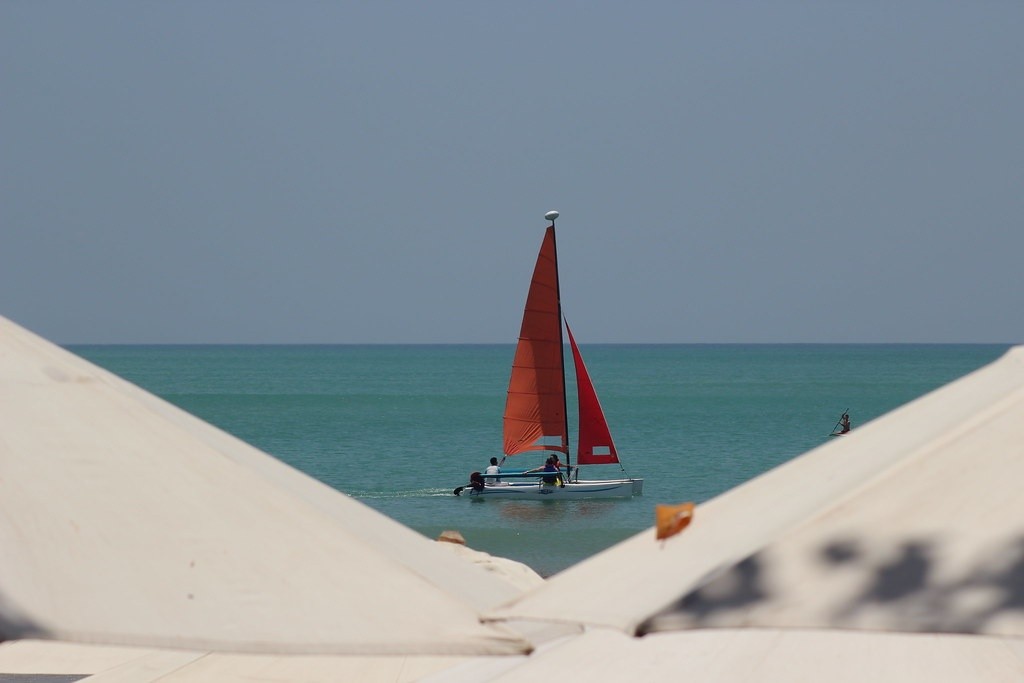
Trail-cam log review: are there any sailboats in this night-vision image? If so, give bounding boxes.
[453,211,645,498]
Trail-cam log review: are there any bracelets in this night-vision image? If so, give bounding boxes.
[527,471,528,473]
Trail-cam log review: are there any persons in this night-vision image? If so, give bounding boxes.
[839,415,850,433]
[523,454,576,486]
[484,457,501,484]
